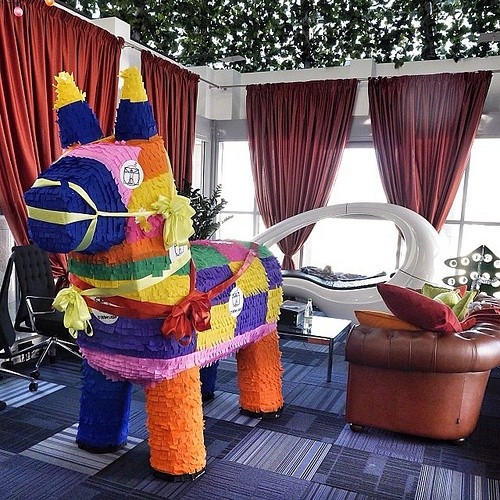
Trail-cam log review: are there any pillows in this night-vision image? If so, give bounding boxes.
[433,290,462,308]
[460,316,477,330]
[421,282,449,298]
[452,289,480,321]
[377,282,464,333]
[455,284,468,295]
[353,309,422,330]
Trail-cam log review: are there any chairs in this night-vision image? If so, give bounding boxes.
[0,243,84,392]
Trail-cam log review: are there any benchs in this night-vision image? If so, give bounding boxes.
[250,201,441,325]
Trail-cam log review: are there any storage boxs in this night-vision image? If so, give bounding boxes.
[278,298,307,326]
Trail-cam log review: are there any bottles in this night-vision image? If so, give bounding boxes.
[303,298,312,329]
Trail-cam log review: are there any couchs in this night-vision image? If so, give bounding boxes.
[344,289,500,446]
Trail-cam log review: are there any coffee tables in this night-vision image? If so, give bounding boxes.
[276,315,352,383]
